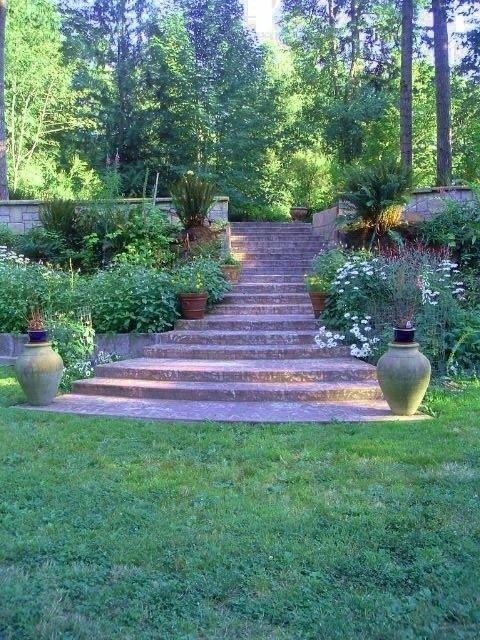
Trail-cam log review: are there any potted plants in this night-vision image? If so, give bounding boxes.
[26,306,48,343]
[177,266,207,321]
[388,301,418,342]
[220,256,241,285]
[309,248,347,319]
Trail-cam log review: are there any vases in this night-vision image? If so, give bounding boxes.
[289,206,309,222]
